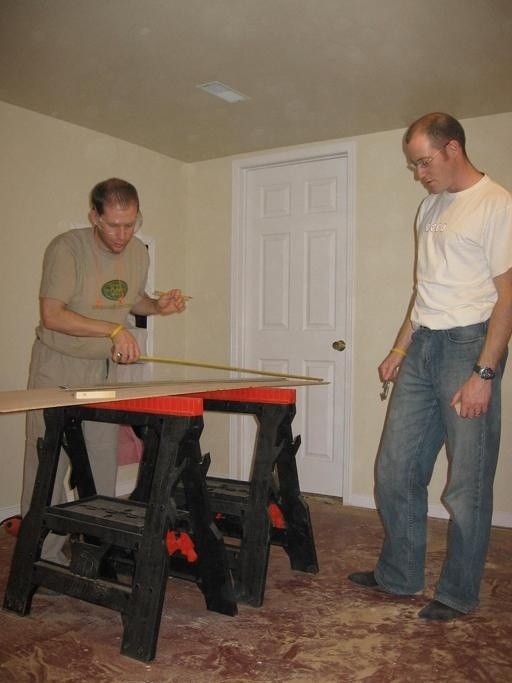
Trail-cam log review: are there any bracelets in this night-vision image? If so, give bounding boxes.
[391,346,406,358]
[110,324,126,340]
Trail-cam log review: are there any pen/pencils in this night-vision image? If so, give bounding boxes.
[153,290,193,302]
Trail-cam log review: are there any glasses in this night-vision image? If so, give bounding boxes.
[93,206,144,236]
[406,141,448,171]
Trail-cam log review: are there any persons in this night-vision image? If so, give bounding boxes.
[22,178,187,596]
[348,111,512,621]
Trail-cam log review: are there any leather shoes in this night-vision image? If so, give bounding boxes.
[347,569,378,587]
[416,600,466,620]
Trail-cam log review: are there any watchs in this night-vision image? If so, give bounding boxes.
[473,363,496,380]
